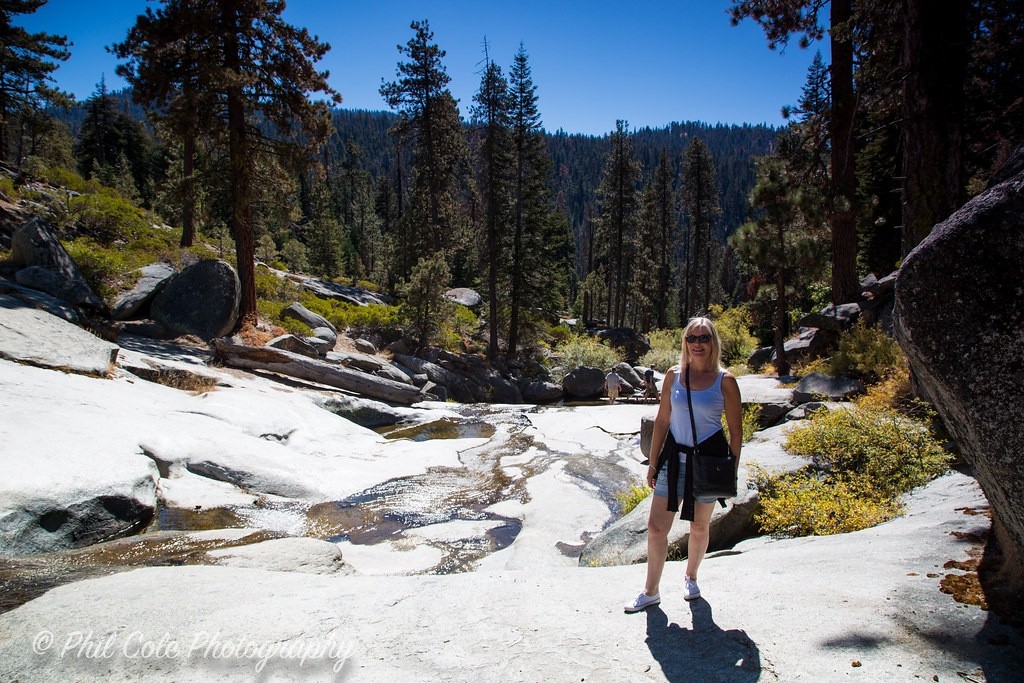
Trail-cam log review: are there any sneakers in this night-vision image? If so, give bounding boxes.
[683,576,701,600]
[623,587,662,612]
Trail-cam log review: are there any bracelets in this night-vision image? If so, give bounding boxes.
[649,465,655,469]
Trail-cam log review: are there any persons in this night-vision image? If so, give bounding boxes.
[605,368,622,404]
[644,364,660,401]
[623,317,744,612]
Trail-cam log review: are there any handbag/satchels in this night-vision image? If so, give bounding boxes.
[691,454,738,498]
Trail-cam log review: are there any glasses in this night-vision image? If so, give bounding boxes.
[685,335,713,343]
